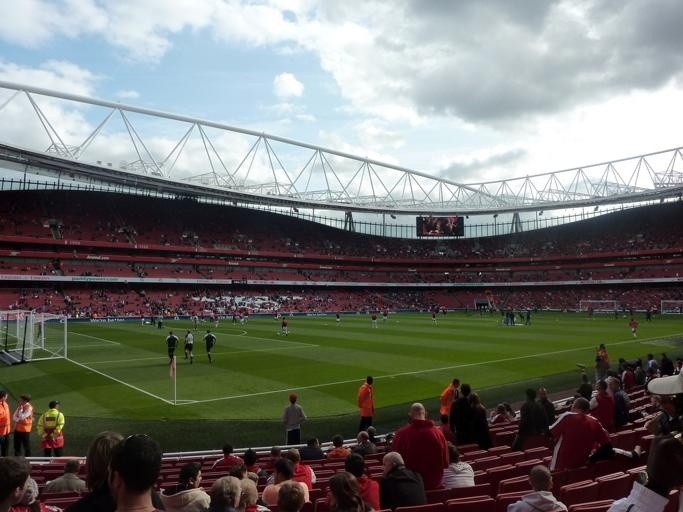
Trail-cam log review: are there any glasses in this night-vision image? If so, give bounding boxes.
[574,394,581,399]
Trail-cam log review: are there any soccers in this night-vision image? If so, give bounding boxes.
[276,332,280,335]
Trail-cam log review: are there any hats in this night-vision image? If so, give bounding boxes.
[576,384,593,401]
[647,365,683,395]
[48,401,59,409]
[289,393,297,401]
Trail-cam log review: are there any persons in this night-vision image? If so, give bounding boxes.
[164,330,178,363]
[594,344,610,378]
[5,217,683,328]
[1,350,682,512]
[281,316,287,336]
[627,317,639,338]
[201,329,216,363]
[182,328,194,364]
